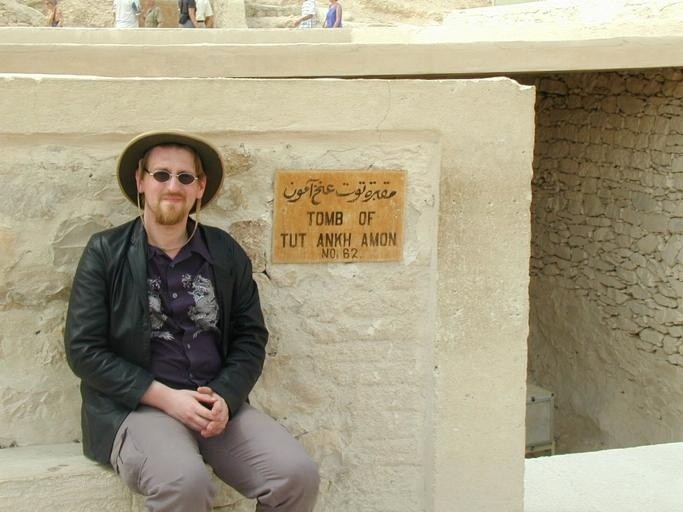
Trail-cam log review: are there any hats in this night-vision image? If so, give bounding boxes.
[117,129,223,212]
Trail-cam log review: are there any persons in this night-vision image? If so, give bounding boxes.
[177,0,200,27]
[112,0,142,28]
[292,0,319,28]
[323,0,342,28]
[143,0,166,27]
[195,0,214,28]
[61,131,320,512]
[45,0,64,28]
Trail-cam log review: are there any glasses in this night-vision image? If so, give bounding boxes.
[144,167,201,185]
